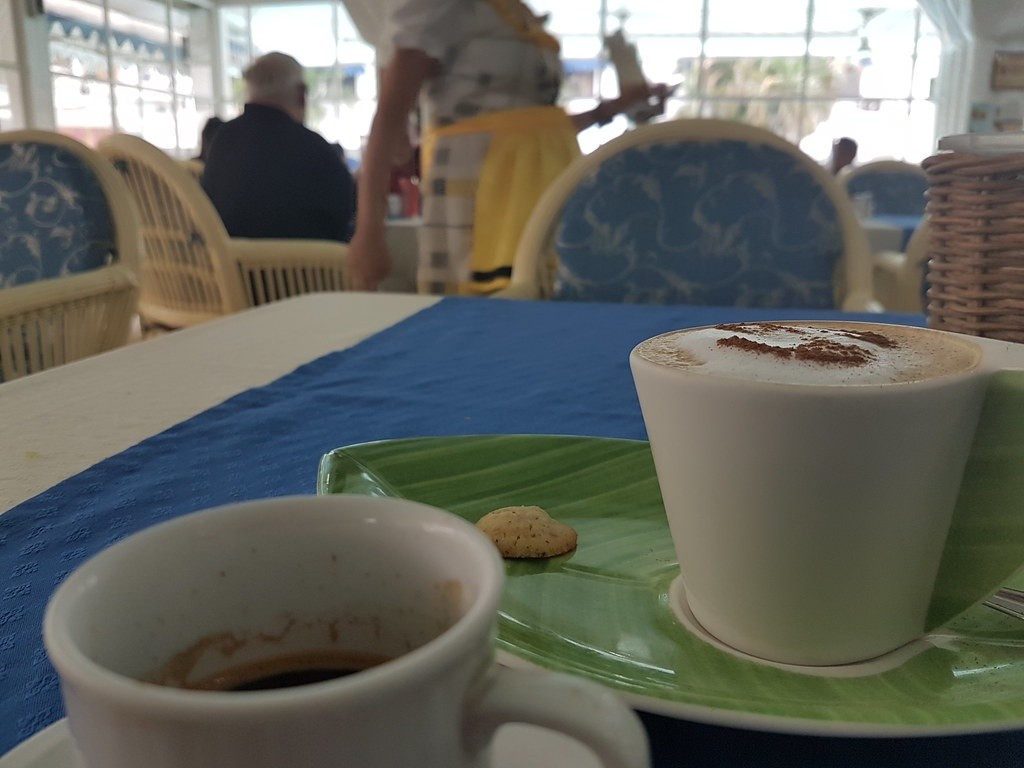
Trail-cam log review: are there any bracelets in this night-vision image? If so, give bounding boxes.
[593,103,613,127]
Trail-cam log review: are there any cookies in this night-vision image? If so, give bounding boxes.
[473,506,578,558]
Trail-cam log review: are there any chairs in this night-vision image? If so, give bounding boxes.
[0,118,938,383]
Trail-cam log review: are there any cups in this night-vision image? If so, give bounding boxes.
[42,493,654,767]
[628,319,985,666]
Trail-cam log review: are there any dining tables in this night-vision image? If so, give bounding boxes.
[0,293,1023,768]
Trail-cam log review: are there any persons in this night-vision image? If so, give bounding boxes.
[191,53,420,305]
[344,0,681,299]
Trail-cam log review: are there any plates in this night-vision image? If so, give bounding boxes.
[311,428,1022,739]
[0,644,605,765]
